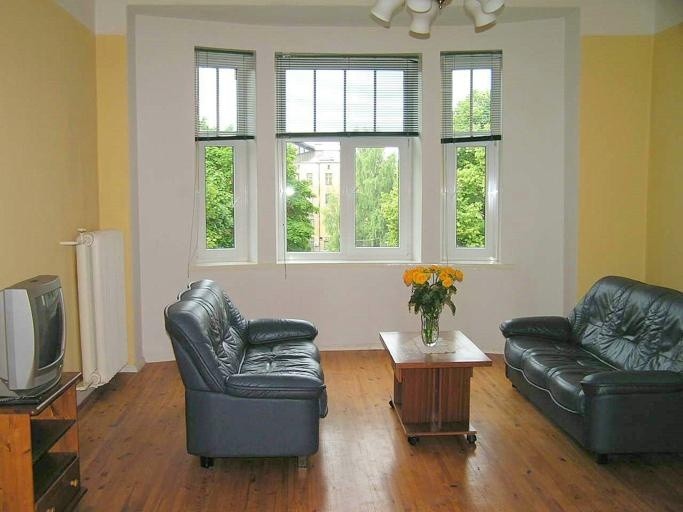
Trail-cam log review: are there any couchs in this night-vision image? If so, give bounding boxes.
[497,272,682,466]
[164,275,331,471]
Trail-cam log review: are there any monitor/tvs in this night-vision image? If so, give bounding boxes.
[0,274,68,399]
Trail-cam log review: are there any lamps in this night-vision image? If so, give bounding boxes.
[366,0,506,41]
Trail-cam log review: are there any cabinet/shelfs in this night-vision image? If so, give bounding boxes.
[0,369,89,512]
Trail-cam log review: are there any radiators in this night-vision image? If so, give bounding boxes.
[55,226,128,394]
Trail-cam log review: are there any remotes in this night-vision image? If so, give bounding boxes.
[0,396,42,405]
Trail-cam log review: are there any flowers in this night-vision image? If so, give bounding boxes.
[399,263,463,344]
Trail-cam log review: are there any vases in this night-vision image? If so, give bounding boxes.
[416,307,442,348]
